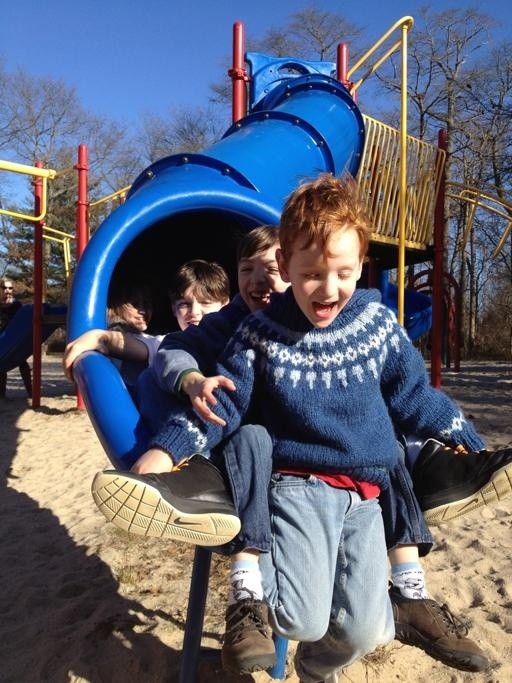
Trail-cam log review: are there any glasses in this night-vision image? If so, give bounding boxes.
[0,286,13,289]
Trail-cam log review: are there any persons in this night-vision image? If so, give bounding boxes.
[62,170,512,683]
[1,275,35,404]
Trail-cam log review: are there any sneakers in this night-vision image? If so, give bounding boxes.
[411,438,512,527]
[389,581,488,672]
[222,592,277,676]
[91,453,241,547]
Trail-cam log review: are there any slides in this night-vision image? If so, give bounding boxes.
[67,51,440,473]
